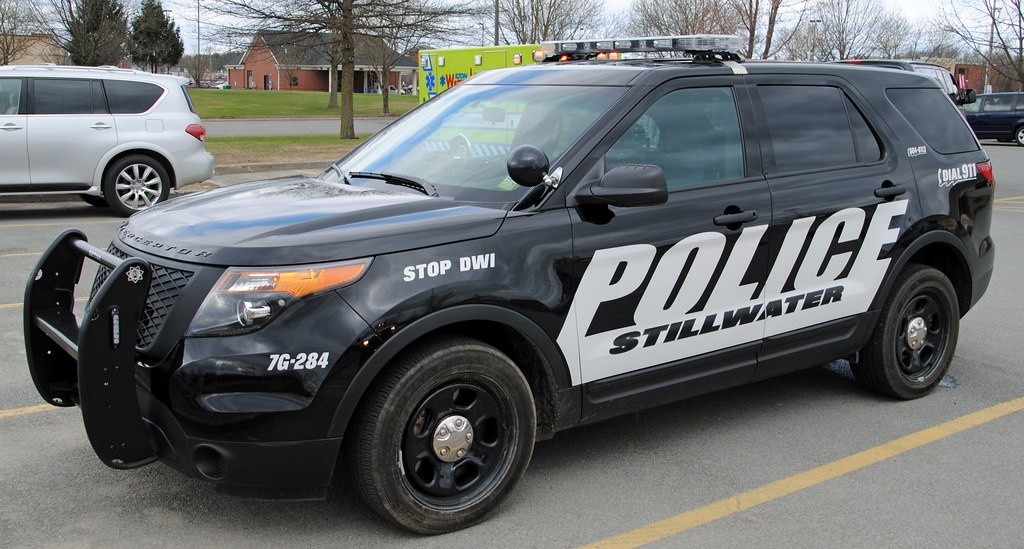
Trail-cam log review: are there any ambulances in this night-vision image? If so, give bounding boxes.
[417,45,658,192]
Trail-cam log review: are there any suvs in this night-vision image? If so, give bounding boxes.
[0,63,214,217]
[23,35,996,536]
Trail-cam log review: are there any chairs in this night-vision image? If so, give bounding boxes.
[515,108,563,159]
[660,105,718,183]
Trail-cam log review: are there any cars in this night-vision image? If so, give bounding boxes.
[963,92,1024,146]
